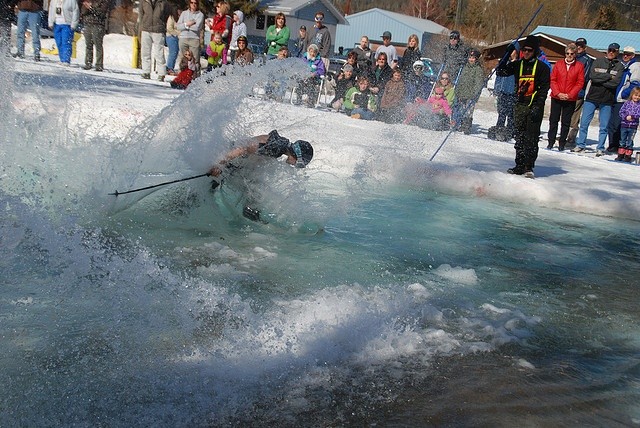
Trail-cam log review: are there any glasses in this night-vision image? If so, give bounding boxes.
[291,143,306,168]
[565,53,574,55]
[450,35,458,39]
[190,2,197,4]
[523,48,534,52]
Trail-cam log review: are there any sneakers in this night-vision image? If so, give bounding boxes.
[571,146,584,152]
[507,164,525,175]
[596,150,606,157]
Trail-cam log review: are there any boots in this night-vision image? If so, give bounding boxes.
[624,149,633,161]
[615,148,626,161]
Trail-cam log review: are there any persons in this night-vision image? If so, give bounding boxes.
[367,51,393,106]
[81,0,108,71]
[455,47,483,134]
[227,10,247,50]
[165,4,181,75]
[303,11,331,57]
[206,31,227,71]
[487,49,517,142]
[170,49,196,89]
[547,42,585,150]
[431,71,455,115]
[344,78,379,119]
[331,63,356,111]
[406,59,431,125]
[379,68,406,123]
[496,34,550,177]
[205,1,233,49]
[399,33,422,78]
[176,0,204,76]
[374,30,399,68]
[156,129,314,225]
[12,0,43,61]
[302,43,326,84]
[47,0,80,68]
[414,87,451,129]
[290,24,306,57]
[564,37,591,146]
[139,0,167,81]
[441,29,469,86]
[333,51,367,83]
[571,42,625,156]
[269,46,289,59]
[234,35,254,66]
[606,45,640,151]
[614,87,640,162]
[351,35,374,66]
[266,12,290,60]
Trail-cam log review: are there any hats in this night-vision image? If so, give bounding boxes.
[608,43,620,51]
[623,46,635,54]
[576,38,586,45]
[413,60,424,71]
[300,26,306,31]
[523,35,539,49]
[380,31,391,37]
[237,36,247,41]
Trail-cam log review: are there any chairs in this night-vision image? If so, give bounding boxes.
[423,64,462,129]
[290,56,330,109]
